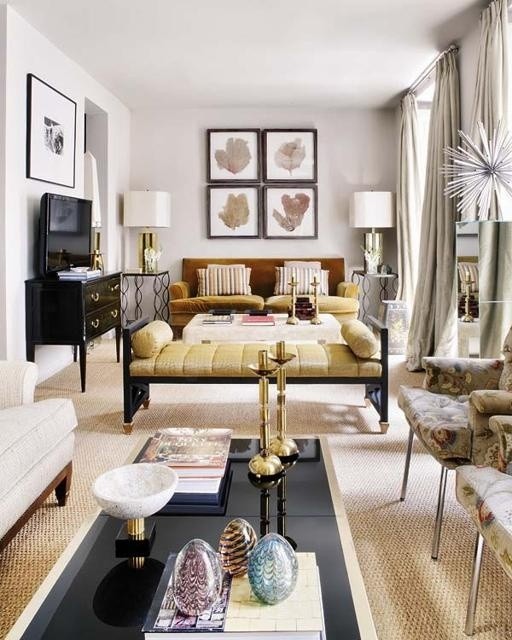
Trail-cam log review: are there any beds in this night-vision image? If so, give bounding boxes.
[122,315,390,435]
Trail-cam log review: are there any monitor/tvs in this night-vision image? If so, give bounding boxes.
[38,192,93,277]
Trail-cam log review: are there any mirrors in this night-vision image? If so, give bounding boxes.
[455,221,479,324]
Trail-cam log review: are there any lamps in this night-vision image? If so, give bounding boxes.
[349,187,396,275]
[122,187,170,273]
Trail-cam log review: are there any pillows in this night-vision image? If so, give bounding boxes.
[196,268,253,296]
[206,264,245,269]
[457,263,479,293]
[273,267,329,296]
[283,260,322,269]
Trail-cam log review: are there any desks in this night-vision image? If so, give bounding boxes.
[120,266,170,336]
[351,269,398,327]
[23,271,122,393]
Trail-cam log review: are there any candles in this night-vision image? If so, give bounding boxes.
[465,270,470,281]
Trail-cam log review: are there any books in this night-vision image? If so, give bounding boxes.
[150,458,233,517]
[139,427,233,493]
[202,315,232,324]
[242,315,275,326]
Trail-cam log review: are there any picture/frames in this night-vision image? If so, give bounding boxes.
[263,184,319,240]
[262,128,318,184]
[25,72,77,190]
[208,127,263,184]
[206,184,263,239]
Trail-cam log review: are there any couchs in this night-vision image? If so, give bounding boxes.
[0,360,79,552]
[166,257,361,342]
[457,255,478,306]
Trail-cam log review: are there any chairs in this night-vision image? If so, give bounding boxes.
[397,327,512,560]
[454,415,512,635]
